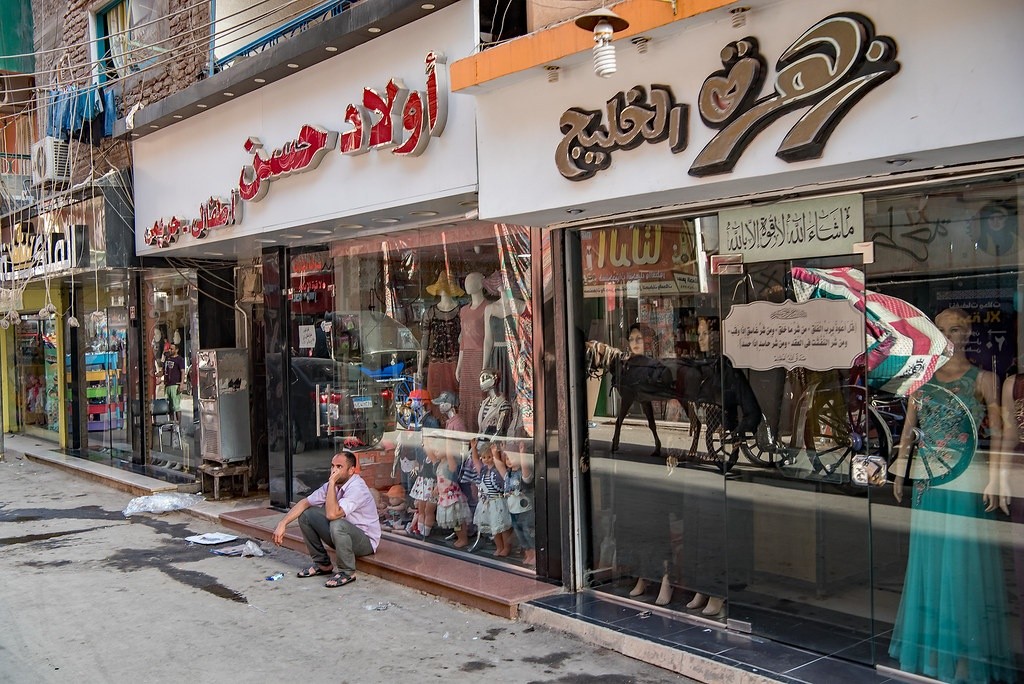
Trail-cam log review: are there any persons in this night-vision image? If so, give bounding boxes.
[26,374,47,427]
[155,344,185,431]
[272,451,381,588]
[612,307,1024,684]
[977,206,1024,257]
[369,271,536,565]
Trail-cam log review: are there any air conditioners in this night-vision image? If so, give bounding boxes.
[29,136,72,190]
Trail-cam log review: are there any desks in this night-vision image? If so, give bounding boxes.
[747,470,902,599]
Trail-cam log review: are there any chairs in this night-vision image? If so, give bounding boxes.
[151,398,182,452]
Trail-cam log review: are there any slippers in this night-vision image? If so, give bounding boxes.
[324,572,356,587]
[297,565,332,578]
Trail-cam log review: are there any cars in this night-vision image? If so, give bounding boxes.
[290,356,398,454]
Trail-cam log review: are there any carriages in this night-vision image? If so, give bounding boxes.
[584,337,978,497]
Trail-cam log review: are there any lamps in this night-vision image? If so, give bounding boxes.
[547,67,558,82]
[730,8,747,28]
[636,39,648,54]
[574,0,629,79]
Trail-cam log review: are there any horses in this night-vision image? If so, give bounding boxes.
[585,341,713,457]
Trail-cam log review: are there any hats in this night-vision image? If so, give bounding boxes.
[382,485,406,501]
[426,270,465,297]
[431,391,460,407]
[409,389,430,404]
[481,269,530,299]
